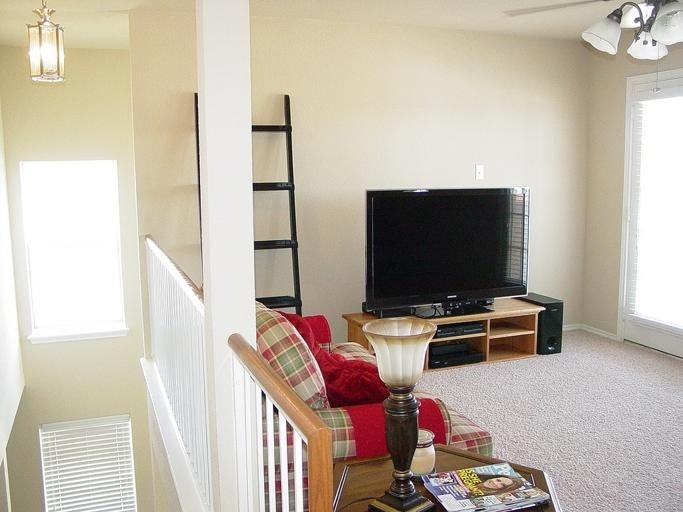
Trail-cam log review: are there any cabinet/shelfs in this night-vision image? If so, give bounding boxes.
[341,297,545,372]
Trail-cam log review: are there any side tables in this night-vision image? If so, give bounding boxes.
[331,443,561,512]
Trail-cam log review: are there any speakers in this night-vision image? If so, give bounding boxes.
[514,292,564,355]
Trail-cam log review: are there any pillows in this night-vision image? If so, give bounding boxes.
[255,299,387,409]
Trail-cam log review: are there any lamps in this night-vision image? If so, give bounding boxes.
[360,317,438,512]
[580,0,682,61]
[24,1,65,83]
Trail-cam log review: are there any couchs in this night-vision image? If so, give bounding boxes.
[195,285,492,512]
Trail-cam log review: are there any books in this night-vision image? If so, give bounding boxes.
[421,461,550,512]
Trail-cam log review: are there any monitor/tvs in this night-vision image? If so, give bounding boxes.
[364,187,530,318]
